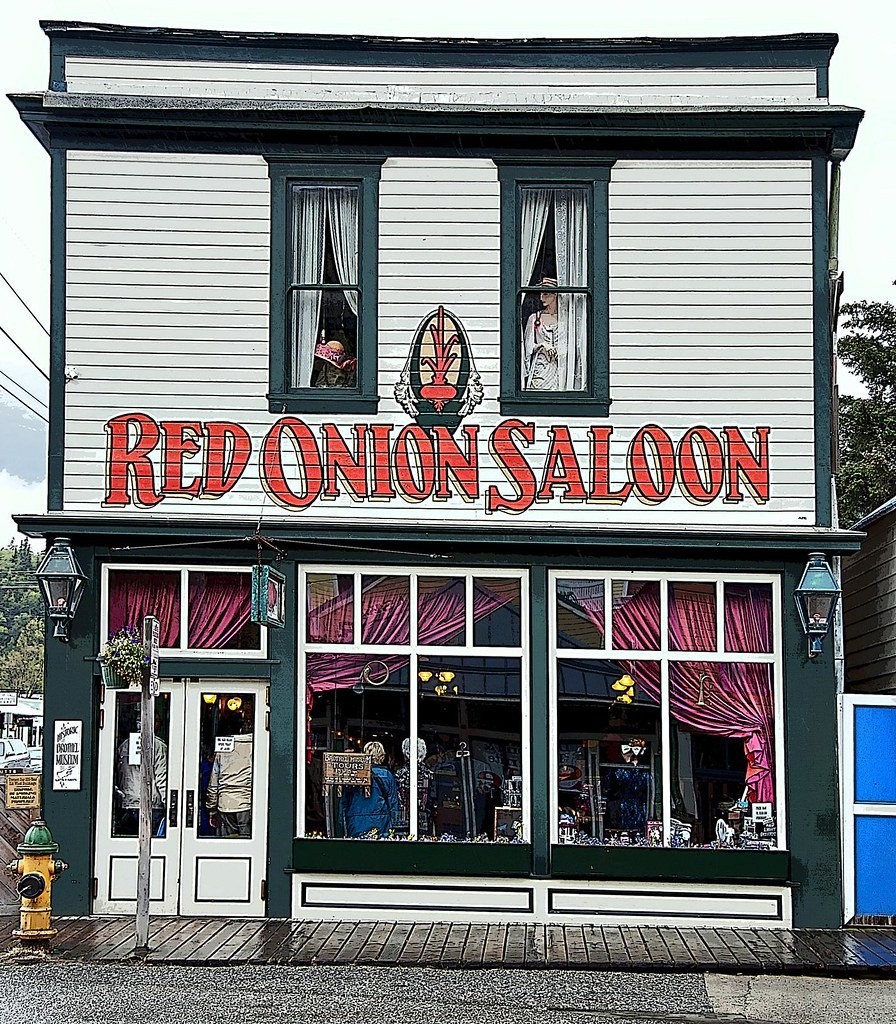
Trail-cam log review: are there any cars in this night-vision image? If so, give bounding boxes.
[27,747,43,774]
[0,739,31,768]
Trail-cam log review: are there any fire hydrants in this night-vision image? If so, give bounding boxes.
[6,818,68,945]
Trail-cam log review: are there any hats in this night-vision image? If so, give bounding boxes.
[314,341,357,371]
[537,278,557,288]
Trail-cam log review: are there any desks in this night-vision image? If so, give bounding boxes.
[493,806,521,841]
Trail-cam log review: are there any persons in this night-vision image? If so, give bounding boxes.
[524,276,561,390]
[601,738,652,839]
[114,708,168,835]
[313,339,357,387]
[337,741,399,839]
[394,736,436,836]
[205,714,254,835]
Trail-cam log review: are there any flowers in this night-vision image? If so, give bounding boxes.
[97,624,150,687]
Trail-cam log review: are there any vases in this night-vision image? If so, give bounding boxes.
[99,662,130,689]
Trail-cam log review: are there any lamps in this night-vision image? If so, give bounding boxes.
[418,671,457,696]
[611,675,635,704]
[792,553,843,659]
[33,537,92,644]
